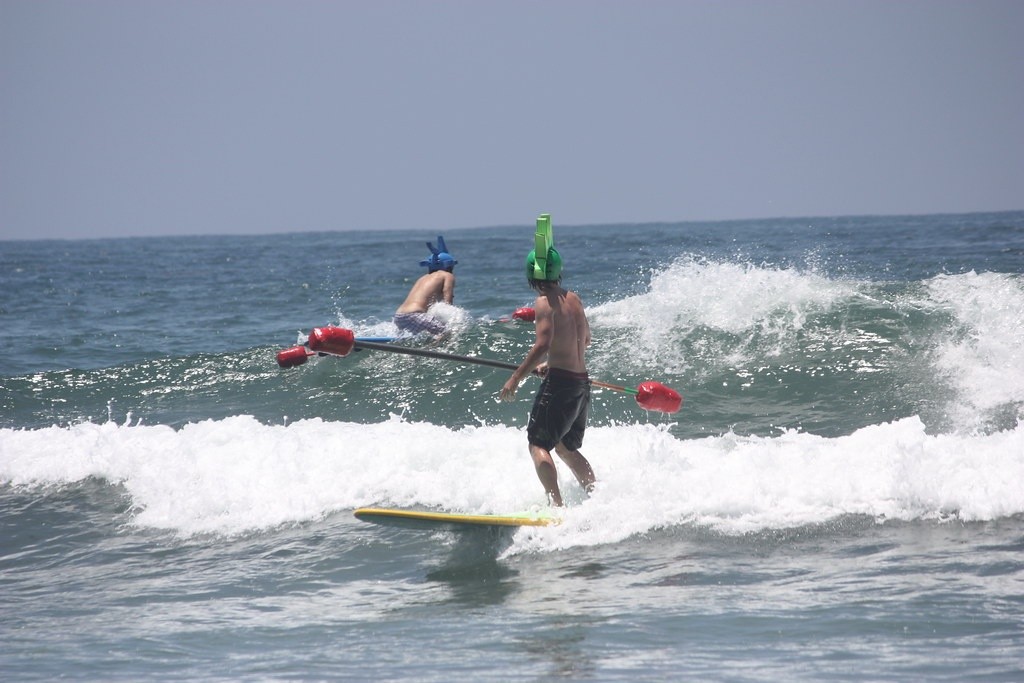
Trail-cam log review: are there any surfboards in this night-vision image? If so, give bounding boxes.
[301,335,399,347]
[352,506,549,532]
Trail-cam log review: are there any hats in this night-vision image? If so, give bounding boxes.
[419,235,458,270]
[526,214,561,281]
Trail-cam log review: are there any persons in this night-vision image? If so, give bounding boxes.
[499,214,599,509]
[394,236,457,339]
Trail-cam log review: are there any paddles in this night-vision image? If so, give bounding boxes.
[276,306,537,369]
[309,325,682,413]
